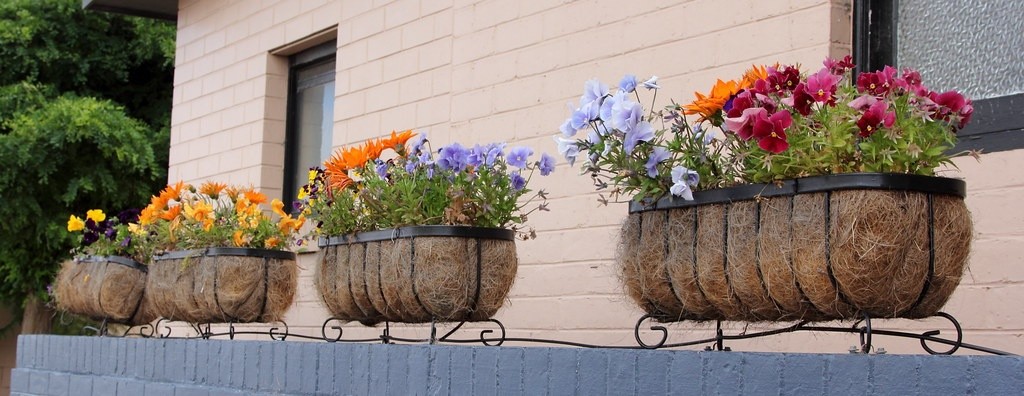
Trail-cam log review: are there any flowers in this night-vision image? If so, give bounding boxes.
[297,131,558,246]
[136,179,299,271]
[67,209,149,266]
[552,55,986,206]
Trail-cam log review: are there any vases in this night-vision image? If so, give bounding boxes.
[146,248,304,340]
[48,254,155,338]
[617,171,974,355]
[314,224,519,347]
